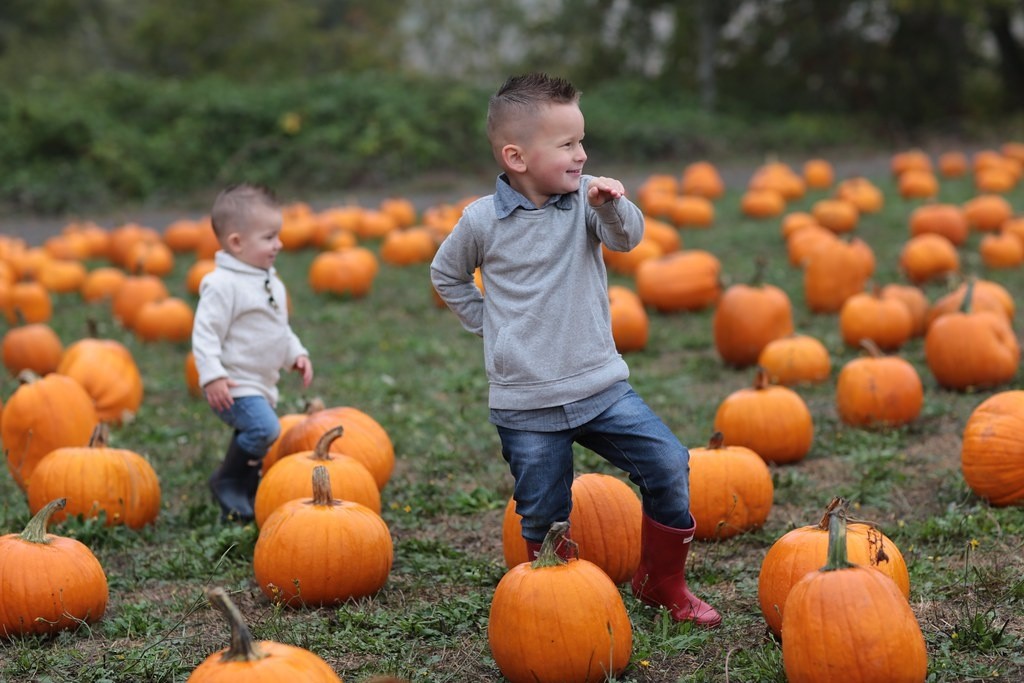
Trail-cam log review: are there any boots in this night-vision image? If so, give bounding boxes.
[631,511,722,628]
[210,429,257,518]
[527,530,569,562]
[220,459,262,523]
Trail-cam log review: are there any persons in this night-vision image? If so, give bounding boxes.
[431,74,722,631]
[192,181,313,521]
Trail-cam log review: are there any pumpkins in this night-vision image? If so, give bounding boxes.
[0,146,1024,683]
